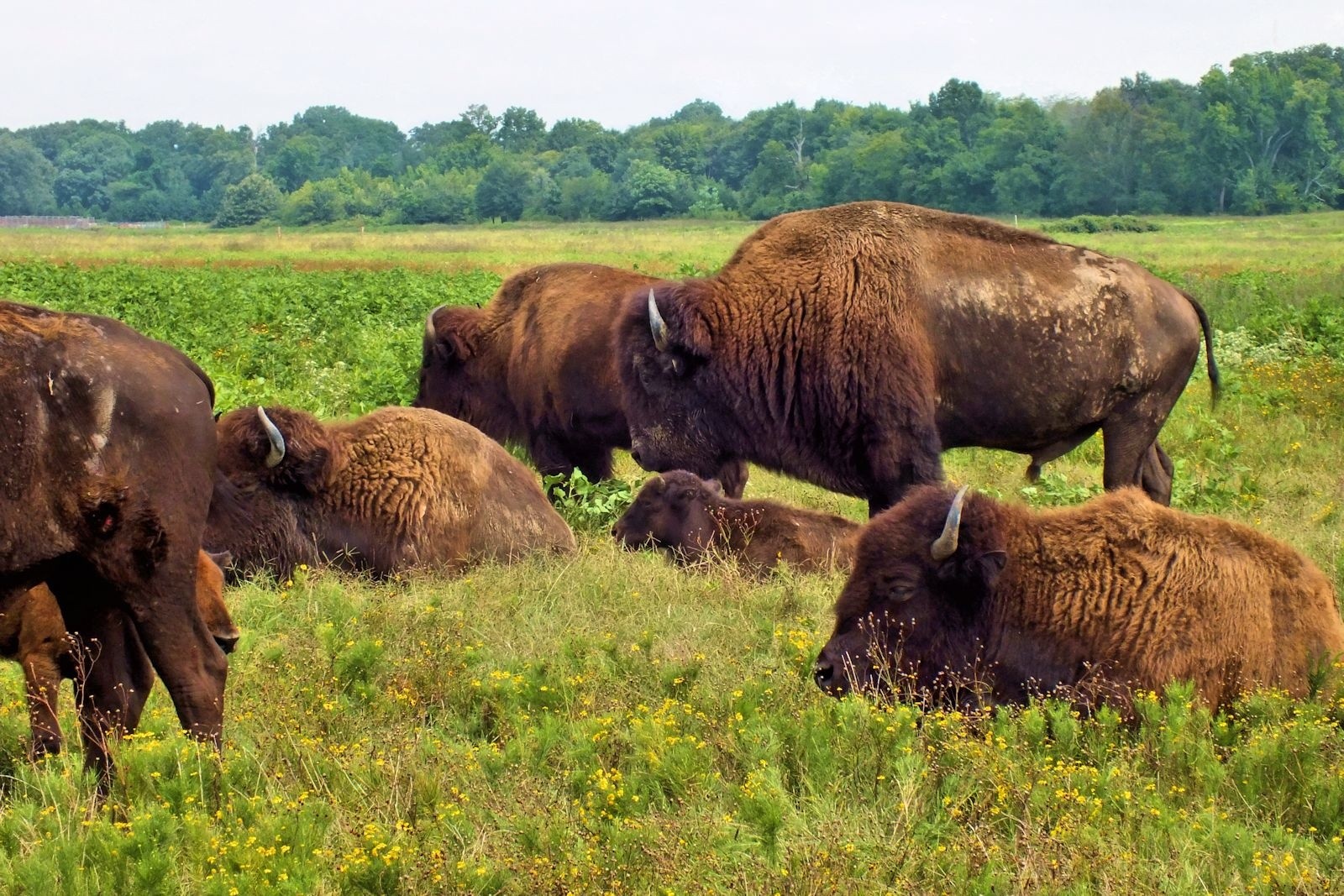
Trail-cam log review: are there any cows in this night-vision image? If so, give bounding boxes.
[611,470,866,576]
[0,302,578,825]
[414,261,673,520]
[611,202,1226,504]
[813,485,1344,721]
